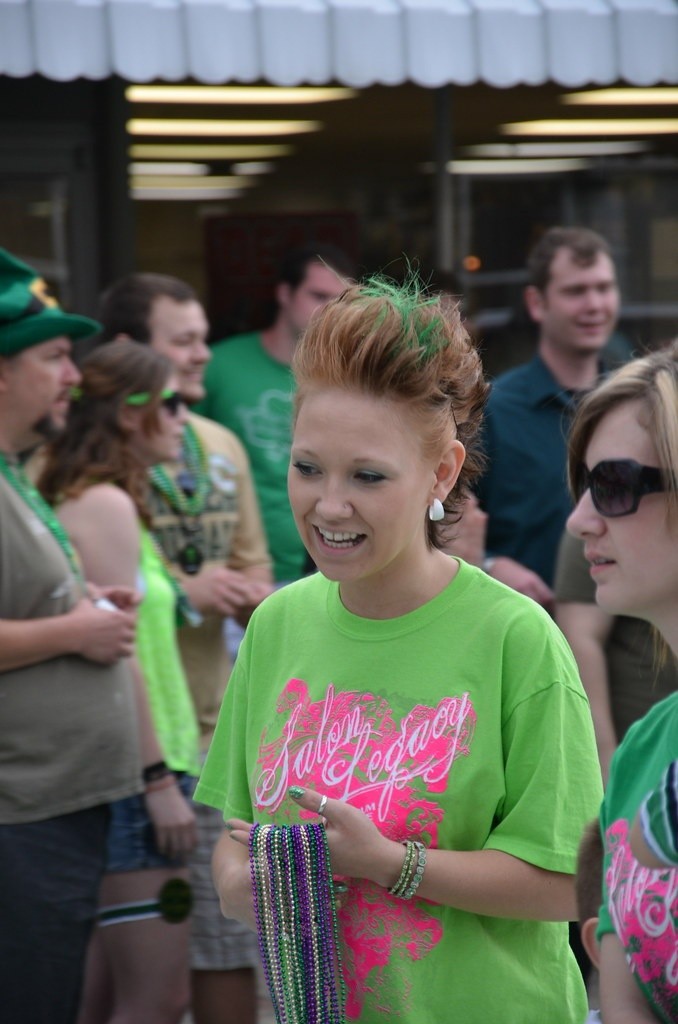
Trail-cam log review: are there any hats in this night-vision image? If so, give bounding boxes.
[0,249,104,356]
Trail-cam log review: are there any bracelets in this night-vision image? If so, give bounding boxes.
[388,841,427,900]
[482,556,496,572]
[143,761,175,793]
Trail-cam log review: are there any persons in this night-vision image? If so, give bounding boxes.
[27,347,207,1024]
[551,506,678,789]
[191,285,603,1024]
[563,342,678,1024]
[105,273,274,1024]
[575,817,603,1024]
[0,250,148,1024]
[193,238,365,589]
[436,226,631,620]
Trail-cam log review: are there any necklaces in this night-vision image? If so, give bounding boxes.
[249,822,346,1023]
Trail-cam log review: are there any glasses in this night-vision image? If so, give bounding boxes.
[575,460,677,517]
[124,389,179,416]
[140,822,194,924]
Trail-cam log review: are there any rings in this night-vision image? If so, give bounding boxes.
[318,795,327,814]
